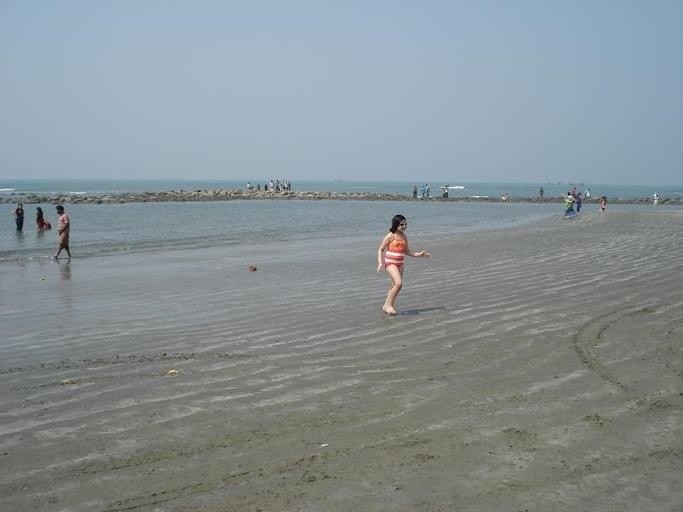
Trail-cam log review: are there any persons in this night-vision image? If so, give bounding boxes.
[53,205,71,259]
[35,207,51,231]
[376,214,430,315]
[536,186,607,219]
[412,184,449,199]
[247,179,291,192]
[12,203,24,232]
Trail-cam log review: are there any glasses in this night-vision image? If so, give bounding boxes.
[400,223,407,226]
